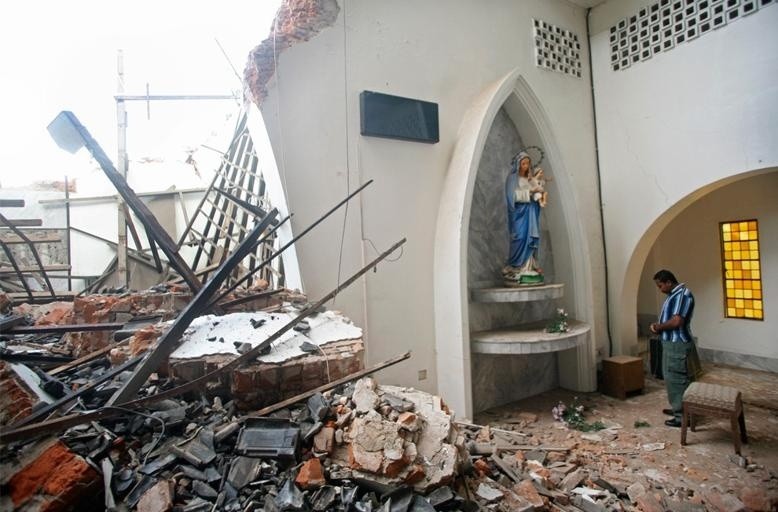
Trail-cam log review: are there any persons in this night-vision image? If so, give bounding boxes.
[504,151,542,274]
[527,166,554,208]
[647,269,695,427]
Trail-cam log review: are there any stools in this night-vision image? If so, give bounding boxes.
[680,381,749,455]
[601,354,646,401]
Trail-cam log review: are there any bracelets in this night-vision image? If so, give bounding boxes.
[650,322,657,333]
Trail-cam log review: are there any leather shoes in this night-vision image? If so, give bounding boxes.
[662,408,674,416]
[664,418,691,427]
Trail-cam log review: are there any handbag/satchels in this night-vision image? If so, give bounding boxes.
[649,338,664,380]
